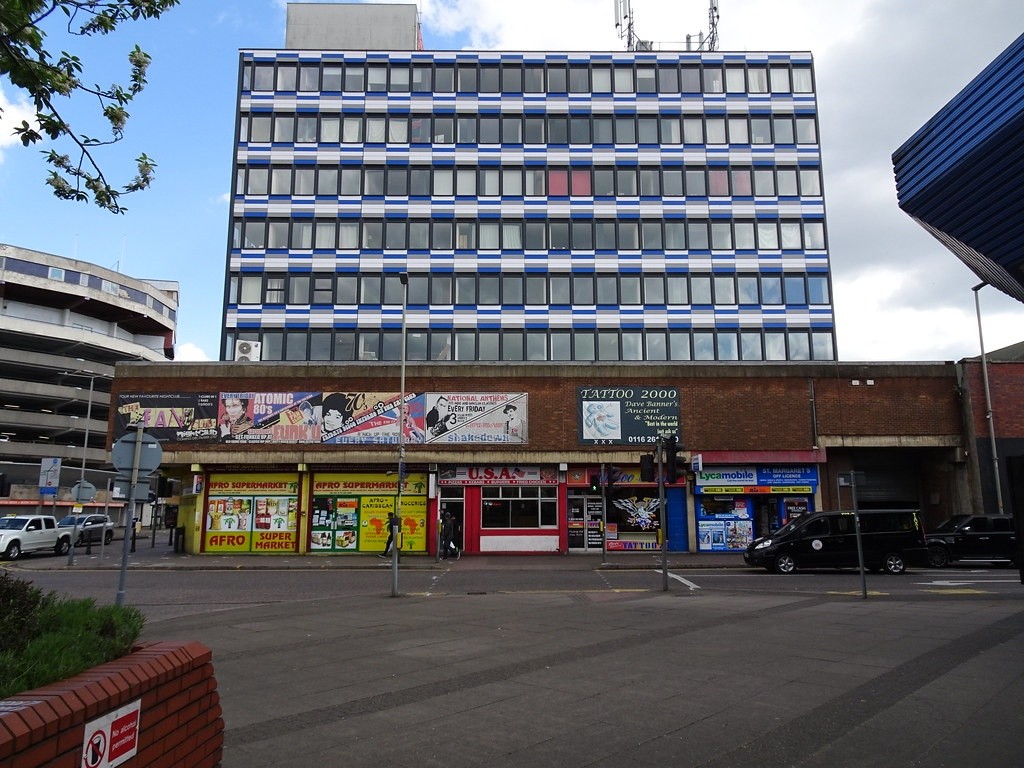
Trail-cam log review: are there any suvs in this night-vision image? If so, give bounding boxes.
[55,514,115,548]
[915,512,1017,570]
[743,507,930,576]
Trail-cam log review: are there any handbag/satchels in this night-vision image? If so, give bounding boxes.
[443,541,455,552]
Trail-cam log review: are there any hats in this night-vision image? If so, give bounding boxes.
[445,512,451,517]
[503,405,517,414]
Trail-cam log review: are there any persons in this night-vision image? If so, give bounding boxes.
[219,399,253,443]
[427,397,451,436]
[440,512,460,560]
[299,401,322,425]
[377,512,398,558]
[503,404,526,443]
[396,403,417,440]
[322,393,354,432]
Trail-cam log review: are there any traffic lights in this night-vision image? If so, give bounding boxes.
[664,434,689,484]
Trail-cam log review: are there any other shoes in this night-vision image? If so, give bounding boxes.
[455,551,460,560]
[377,554,386,559]
[439,556,447,561]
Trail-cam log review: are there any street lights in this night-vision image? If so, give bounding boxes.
[392,270,410,598]
[973,281,1004,516]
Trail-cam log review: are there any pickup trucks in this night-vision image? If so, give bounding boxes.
[0,514,82,561]
